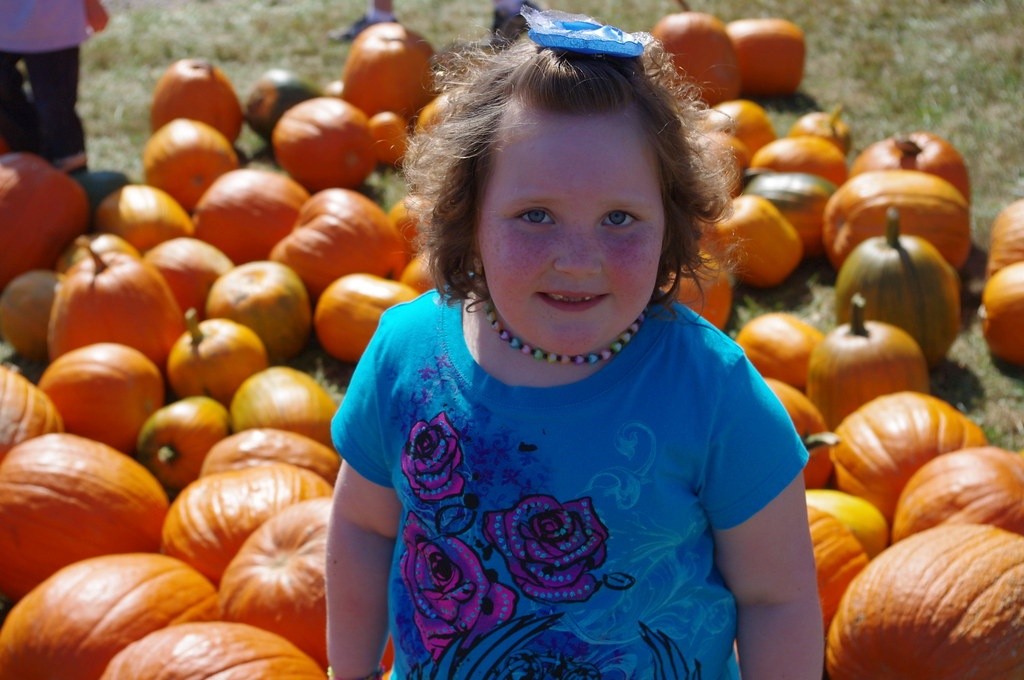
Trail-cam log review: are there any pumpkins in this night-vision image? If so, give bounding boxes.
[0,0,1024,680]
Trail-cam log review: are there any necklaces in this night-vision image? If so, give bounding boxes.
[484,300,648,364]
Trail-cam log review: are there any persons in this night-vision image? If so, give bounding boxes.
[0,0,110,173]
[325,4,828,680]
